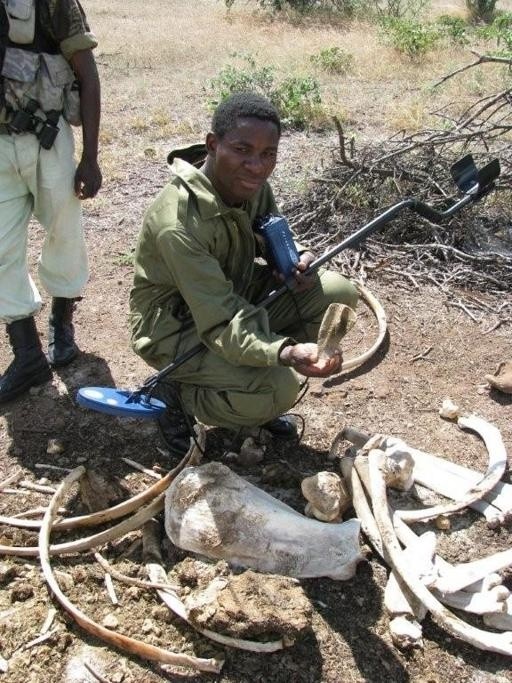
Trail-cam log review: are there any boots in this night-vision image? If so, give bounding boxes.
[144,376,197,455]
[47,297,78,367]
[258,416,299,439]
[0,315,53,403]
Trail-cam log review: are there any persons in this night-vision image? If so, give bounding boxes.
[130,94,358,456]
[0,0,102,400]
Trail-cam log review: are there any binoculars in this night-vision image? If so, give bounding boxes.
[9,100,59,150]
